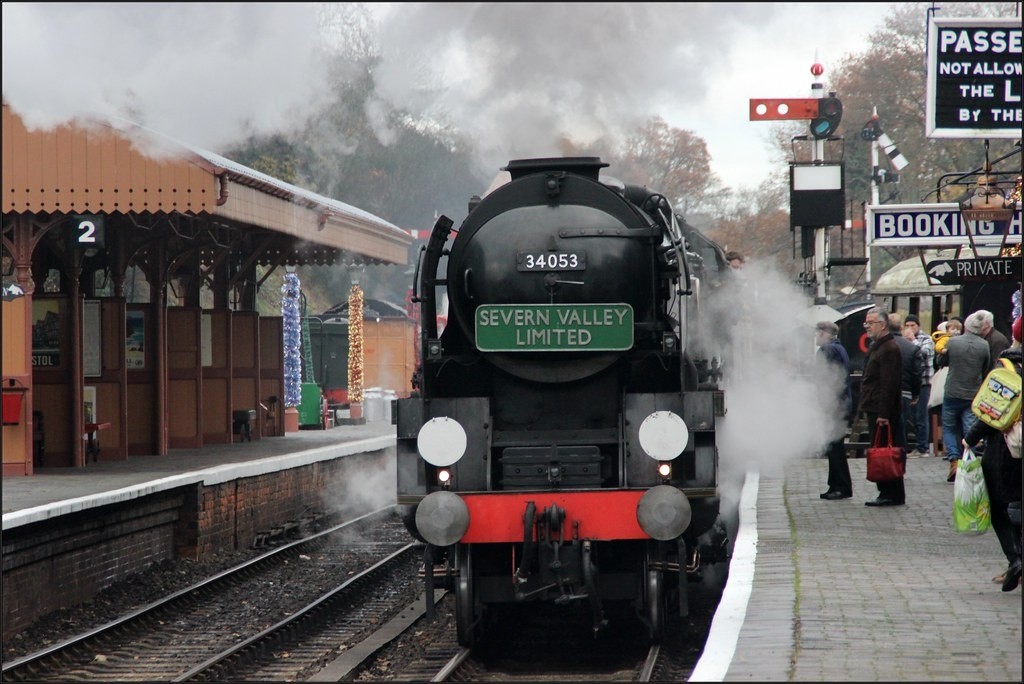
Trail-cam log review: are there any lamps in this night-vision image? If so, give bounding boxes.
[959,143,1017,258]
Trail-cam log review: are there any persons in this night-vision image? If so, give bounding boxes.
[727,248,1022,591]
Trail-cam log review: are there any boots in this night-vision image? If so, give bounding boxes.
[947,460,957,482]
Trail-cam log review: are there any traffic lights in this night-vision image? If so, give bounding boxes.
[811,97,845,141]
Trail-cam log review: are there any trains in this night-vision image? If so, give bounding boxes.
[390,155,742,646]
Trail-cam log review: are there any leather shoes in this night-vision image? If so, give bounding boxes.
[866,497,905,506]
[820,487,833,498]
[827,490,852,499]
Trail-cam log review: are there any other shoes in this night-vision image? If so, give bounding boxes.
[992,571,1022,584]
[1002,559,1022,592]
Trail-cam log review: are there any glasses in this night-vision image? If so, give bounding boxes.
[863,321,882,327]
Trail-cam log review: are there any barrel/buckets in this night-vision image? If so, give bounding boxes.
[2,378,24,424]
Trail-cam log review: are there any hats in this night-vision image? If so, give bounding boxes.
[964,312,986,334]
[1013,316,1022,343]
[905,314,920,326]
[817,321,839,335]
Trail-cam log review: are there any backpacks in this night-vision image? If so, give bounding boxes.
[971,358,1022,432]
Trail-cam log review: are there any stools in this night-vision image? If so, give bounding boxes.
[233,409,256,442]
[86,422,112,462]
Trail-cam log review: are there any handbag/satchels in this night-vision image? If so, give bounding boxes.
[954,448,991,537]
[867,423,906,483]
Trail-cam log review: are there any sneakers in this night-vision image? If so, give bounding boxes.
[906,449,929,459]
